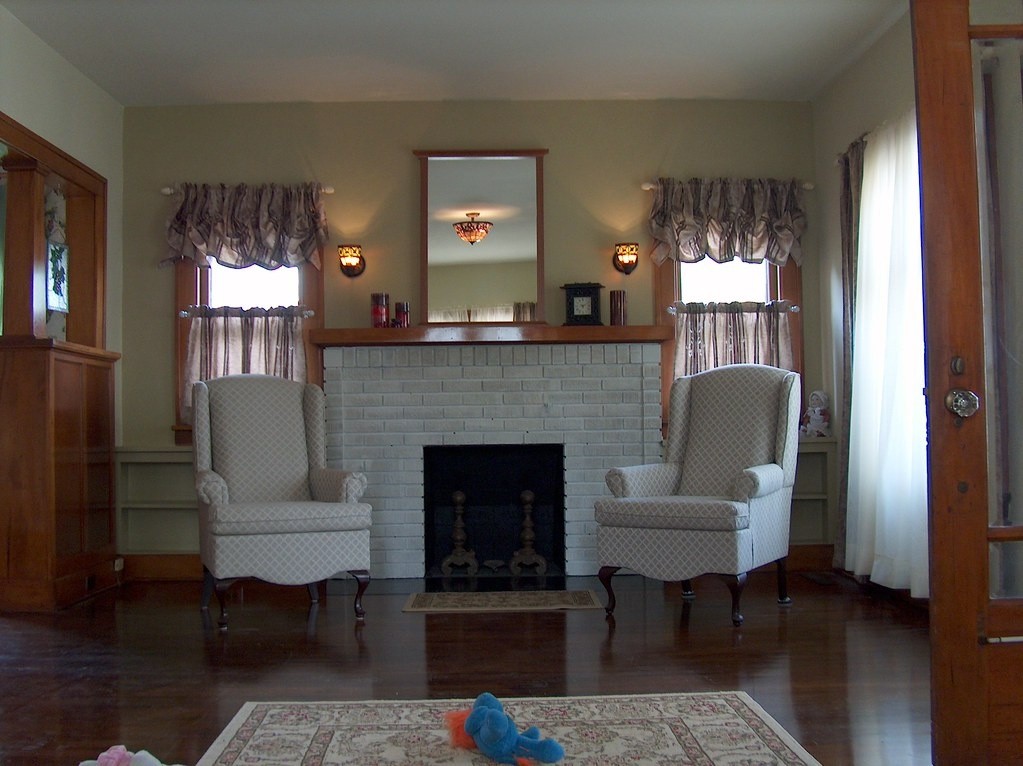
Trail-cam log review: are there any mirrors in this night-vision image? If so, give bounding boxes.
[412,147,550,325]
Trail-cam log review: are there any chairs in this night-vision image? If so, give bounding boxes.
[190,374,373,634]
[591,363,802,628]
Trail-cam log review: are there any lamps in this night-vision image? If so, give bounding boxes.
[452,213,494,246]
[613,242,640,276]
[337,244,366,277]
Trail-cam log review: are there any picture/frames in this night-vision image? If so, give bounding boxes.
[47,241,69,313]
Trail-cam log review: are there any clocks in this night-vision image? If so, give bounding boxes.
[559,281,605,326]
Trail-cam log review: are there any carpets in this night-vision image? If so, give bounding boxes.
[400,589,604,612]
[193,690,823,766]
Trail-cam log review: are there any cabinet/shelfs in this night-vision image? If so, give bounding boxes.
[113,446,203,581]
[766,437,839,574]
[0,337,122,615]
